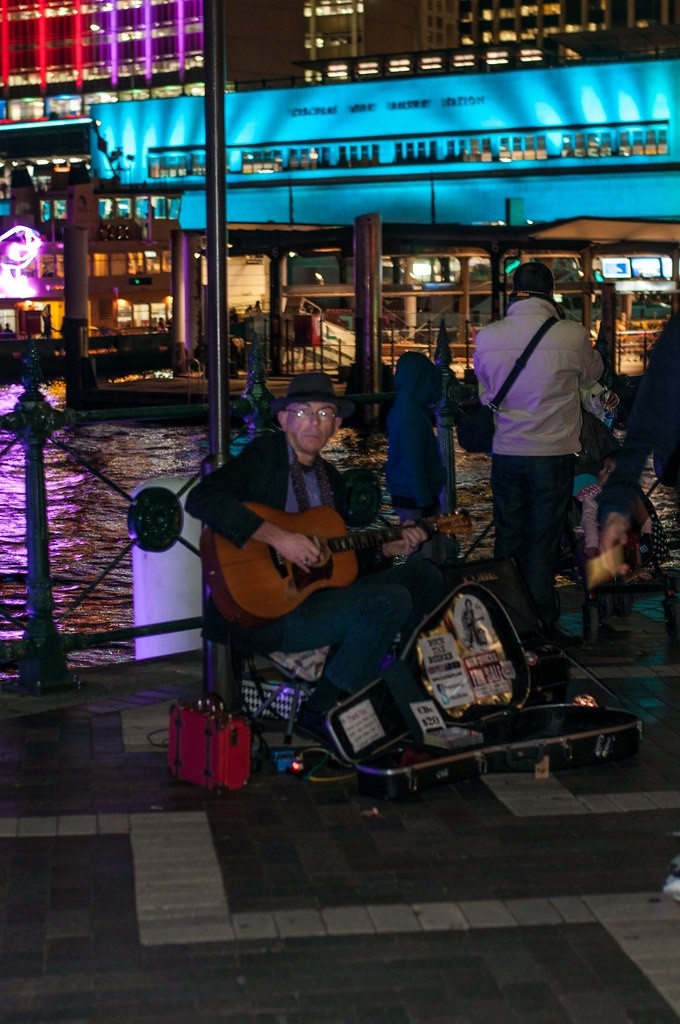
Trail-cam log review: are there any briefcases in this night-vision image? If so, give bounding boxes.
[167,695,253,797]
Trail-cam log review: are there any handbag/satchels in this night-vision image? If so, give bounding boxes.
[454,402,497,455]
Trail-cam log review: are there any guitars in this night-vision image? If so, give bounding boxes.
[202,501,473,624]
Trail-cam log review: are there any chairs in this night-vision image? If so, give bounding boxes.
[199,526,332,746]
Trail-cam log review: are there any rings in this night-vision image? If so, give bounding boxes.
[302,558,307,565]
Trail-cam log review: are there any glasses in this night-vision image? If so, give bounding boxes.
[282,407,337,419]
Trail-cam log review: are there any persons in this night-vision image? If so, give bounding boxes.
[594,307,680,574]
[473,263,604,649]
[577,451,653,583]
[411,546,417,551]
[578,382,620,420]
[386,351,442,526]
[184,373,444,758]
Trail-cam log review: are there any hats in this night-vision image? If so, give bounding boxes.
[267,372,356,419]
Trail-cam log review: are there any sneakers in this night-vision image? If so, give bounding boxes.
[292,700,336,751]
